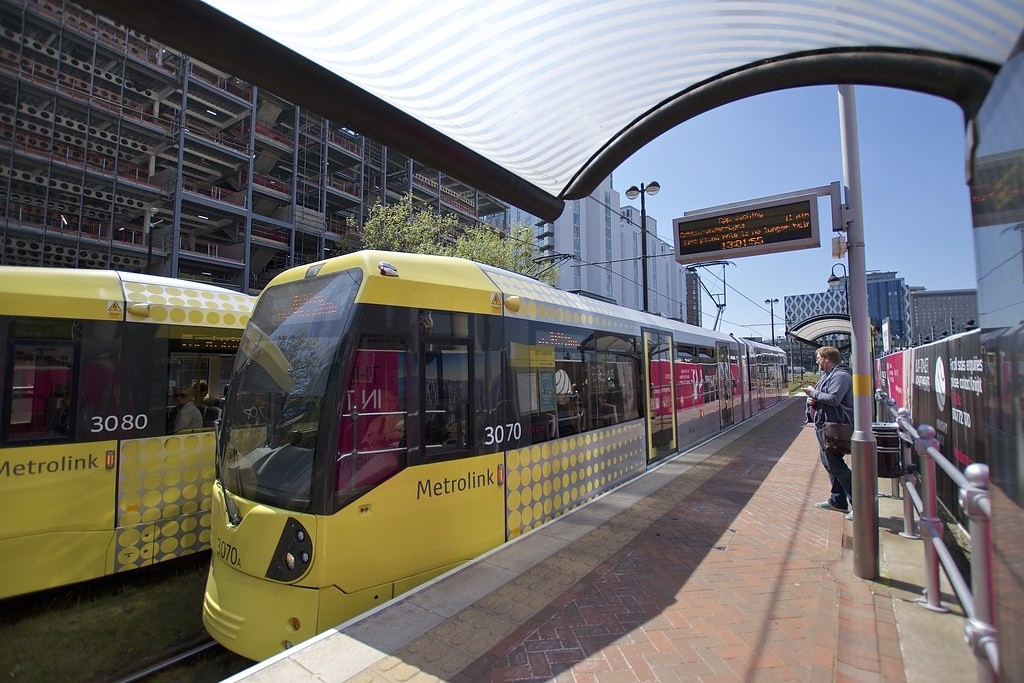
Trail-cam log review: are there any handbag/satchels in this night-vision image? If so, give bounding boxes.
[821,422,854,454]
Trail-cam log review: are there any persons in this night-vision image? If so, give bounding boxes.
[172,376,208,432]
[800,346,854,521]
[361,389,405,451]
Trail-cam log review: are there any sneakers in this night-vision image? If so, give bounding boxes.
[814,498,849,512]
[845,510,853,521]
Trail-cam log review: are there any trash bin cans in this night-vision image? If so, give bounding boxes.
[872,423,900,478]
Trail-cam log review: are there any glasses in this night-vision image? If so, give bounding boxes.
[174,393,187,399]
[815,356,826,362]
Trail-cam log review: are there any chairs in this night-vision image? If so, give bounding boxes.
[196,397,224,427]
[548,403,619,438]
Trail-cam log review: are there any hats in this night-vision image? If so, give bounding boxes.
[48,346,74,363]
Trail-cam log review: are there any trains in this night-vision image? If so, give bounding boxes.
[202,250,788,662]
[0,266,258,599]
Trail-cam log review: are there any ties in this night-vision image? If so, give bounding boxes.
[377,417,386,434]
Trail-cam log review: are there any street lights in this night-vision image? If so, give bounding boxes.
[626,181,661,312]
[765,299,779,347]
[777,335,781,346]
[827,262,851,353]
